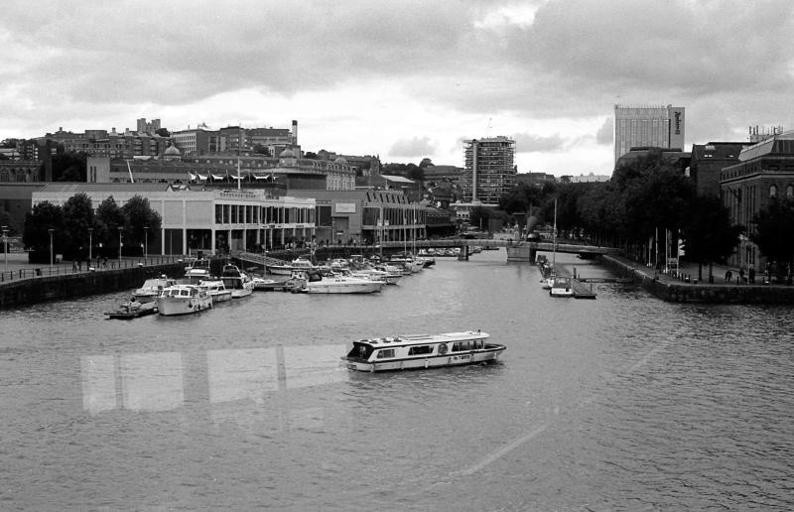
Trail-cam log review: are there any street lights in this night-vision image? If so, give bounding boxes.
[262,225,267,256]
[2,225,149,281]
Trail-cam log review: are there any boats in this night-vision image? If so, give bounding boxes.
[339,330,507,373]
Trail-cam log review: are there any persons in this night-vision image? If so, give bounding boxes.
[724,264,756,283]
[285,237,370,249]
[73,254,108,271]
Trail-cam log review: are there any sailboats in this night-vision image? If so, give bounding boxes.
[536,199,573,296]
